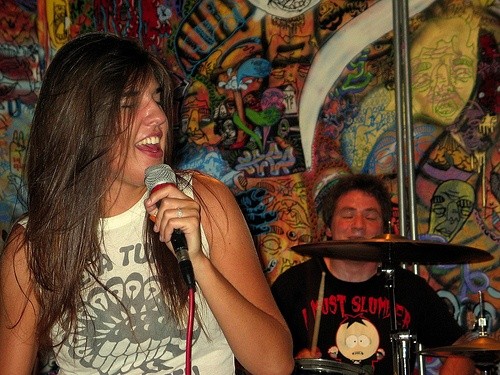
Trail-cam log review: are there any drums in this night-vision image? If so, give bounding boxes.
[294,355,373,375]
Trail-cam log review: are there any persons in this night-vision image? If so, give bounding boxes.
[270,175,475,375]
[0,33,295,375]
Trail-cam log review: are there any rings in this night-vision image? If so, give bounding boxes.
[177,208,183,219]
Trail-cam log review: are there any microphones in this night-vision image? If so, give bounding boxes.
[144,163,196,288]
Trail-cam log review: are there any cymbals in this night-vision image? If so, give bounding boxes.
[291,233,494,264]
[413,329,500,361]
